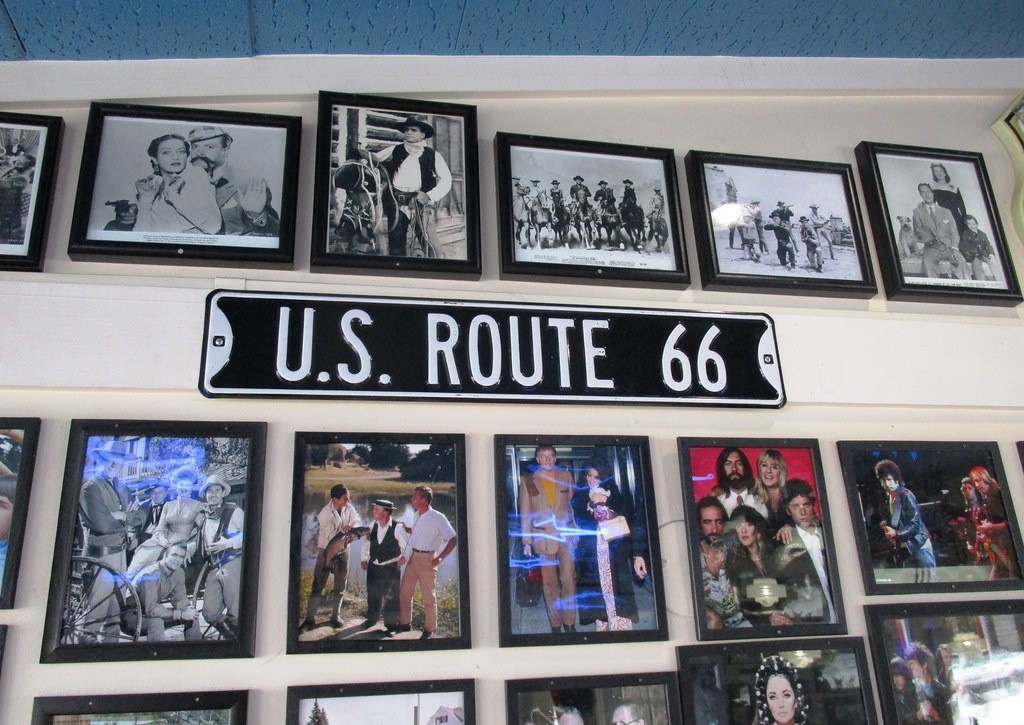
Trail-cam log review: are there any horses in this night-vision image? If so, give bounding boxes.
[329,148,438,258]
[513,184,669,254]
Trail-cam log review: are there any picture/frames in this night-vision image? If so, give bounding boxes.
[863,599,1024,725]
[677,436,848,642]
[287,431,472,654]
[503,636,878,725]
[286,678,477,725]
[38,419,267,666]
[0,417,42,610]
[68,101,303,270]
[836,440,1024,597]
[493,433,670,649]
[0,110,66,273]
[493,131,692,285]
[30,690,249,725]
[684,149,878,301]
[308,88,483,275]
[854,140,1024,309]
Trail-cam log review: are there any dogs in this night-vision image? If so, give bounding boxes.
[102,198,139,230]
[896,215,922,261]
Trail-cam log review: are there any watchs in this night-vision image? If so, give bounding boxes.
[439,558,442,562]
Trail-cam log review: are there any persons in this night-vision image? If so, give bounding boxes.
[363,112,452,258]
[0,138,34,226]
[519,449,639,629]
[914,163,1004,284]
[874,459,937,569]
[552,707,584,725]
[955,469,1022,578]
[696,442,838,629]
[752,655,807,725]
[0,473,19,585]
[508,175,665,228]
[611,700,650,725]
[893,644,962,725]
[727,197,838,272]
[81,442,244,641]
[309,483,457,640]
[107,124,279,237]
